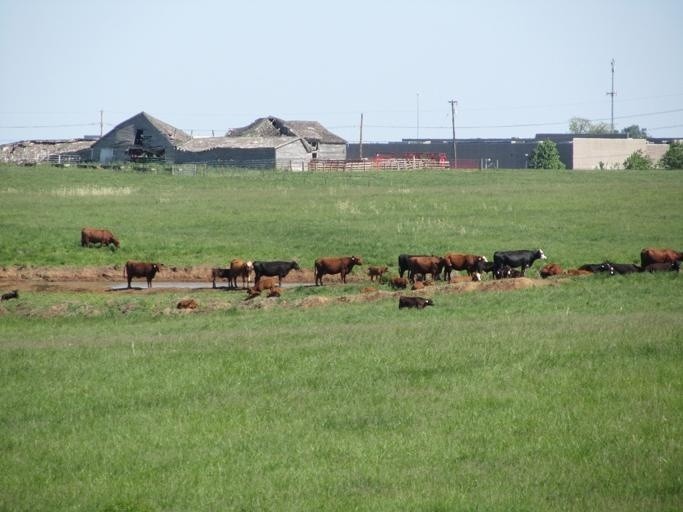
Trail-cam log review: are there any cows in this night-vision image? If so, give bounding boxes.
[412,279,433,290]
[367,265,389,282]
[122,261,164,289]
[398,295,433,311]
[211,247,300,302]
[641,244,683,269]
[314,255,363,286]
[176,299,198,309]
[81,227,121,249]
[398,248,563,284]
[2,289,18,301]
[387,277,410,290]
[564,260,683,275]
